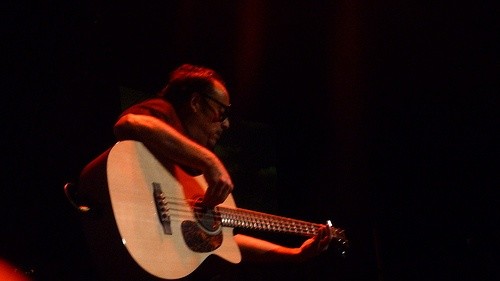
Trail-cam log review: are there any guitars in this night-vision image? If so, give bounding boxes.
[73,138,349,281]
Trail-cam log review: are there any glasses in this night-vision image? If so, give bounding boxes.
[209,94,234,120]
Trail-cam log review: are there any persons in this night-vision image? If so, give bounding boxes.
[113,64,335,281]
